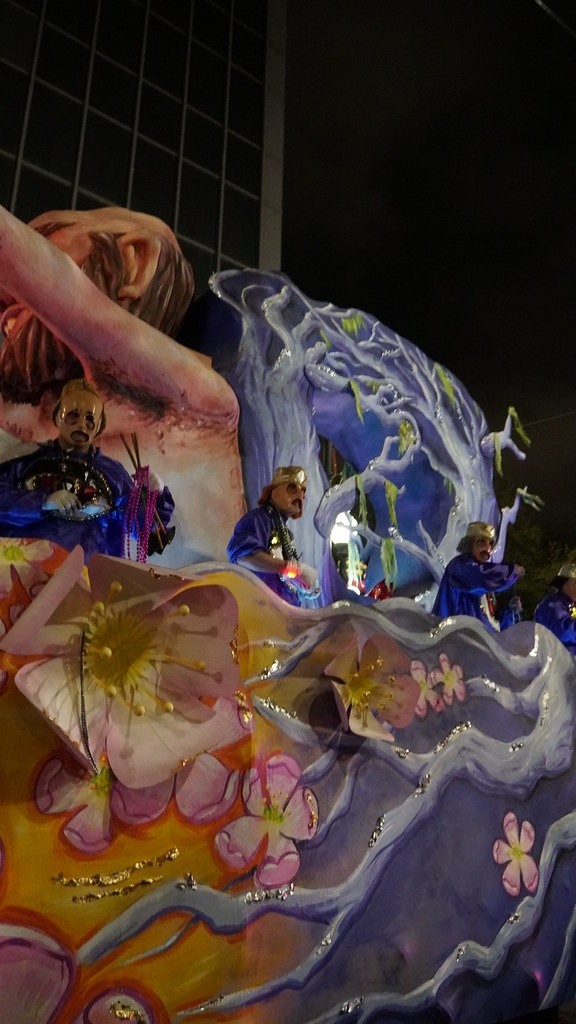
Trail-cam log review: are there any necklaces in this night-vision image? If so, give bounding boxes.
[122,465,159,562]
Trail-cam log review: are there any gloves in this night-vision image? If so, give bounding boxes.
[286,561,322,600]
[131,471,165,495]
[42,490,82,517]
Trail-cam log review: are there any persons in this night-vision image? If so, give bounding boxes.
[0,207,245,572]
[0,378,176,563]
[430,521,525,627]
[533,561,576,656]
[499,596,522,631]
[227,466,322,607]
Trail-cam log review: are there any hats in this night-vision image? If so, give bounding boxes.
[271,466,307,487]
[465,521,496,542]
[557,562,576,578]
